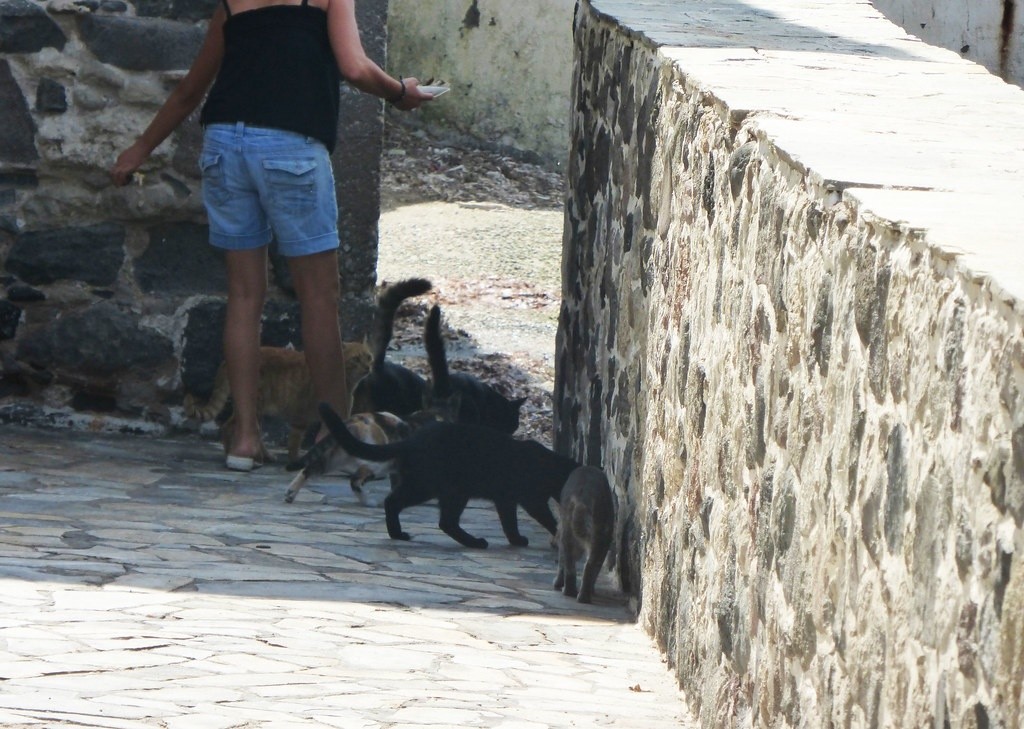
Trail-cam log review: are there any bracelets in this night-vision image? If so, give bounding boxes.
[386,76,405,108]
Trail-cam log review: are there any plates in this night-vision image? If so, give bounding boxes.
[417,85,450,97]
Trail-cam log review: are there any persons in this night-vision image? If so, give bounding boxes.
[111,0,437,471]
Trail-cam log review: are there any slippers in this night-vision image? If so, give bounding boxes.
[225,453,278,469]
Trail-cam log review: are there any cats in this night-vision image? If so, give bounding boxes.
[182,278,614,602]
